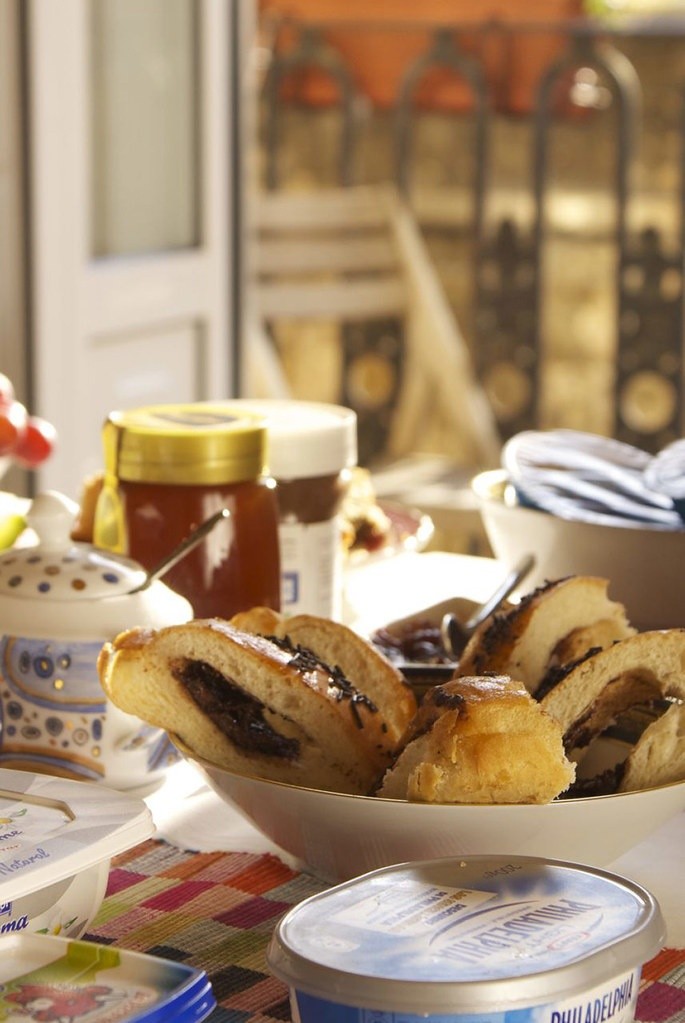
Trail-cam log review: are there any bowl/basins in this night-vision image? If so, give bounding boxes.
[471,467,684,630]
[164,728,685,870]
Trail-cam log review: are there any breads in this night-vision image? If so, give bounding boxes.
[95,575,684,803]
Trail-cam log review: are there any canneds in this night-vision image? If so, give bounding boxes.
[83,392,356,631]
